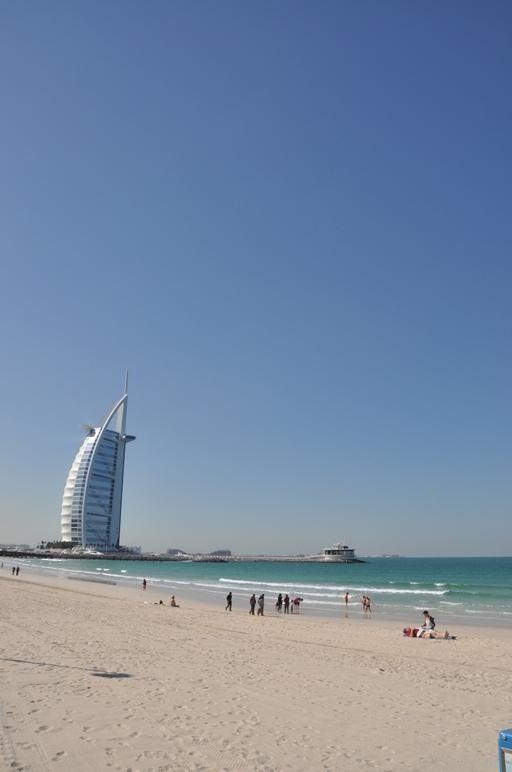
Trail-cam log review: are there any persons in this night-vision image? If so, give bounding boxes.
[224,589,234,612]
[277,592,282,612]
[282,593,290,615]
[293,597,304,614]
[344,591,350,606]
[248,593,256,615]
[365,596,372,613]
[142,577,147,592]
[421,609,435,630]
[11,566,16,575]
[290,598,293,614]
[403,626,449,639]
[361,595,366,610]
[257,593,265,616]
[16,565,21,576]
